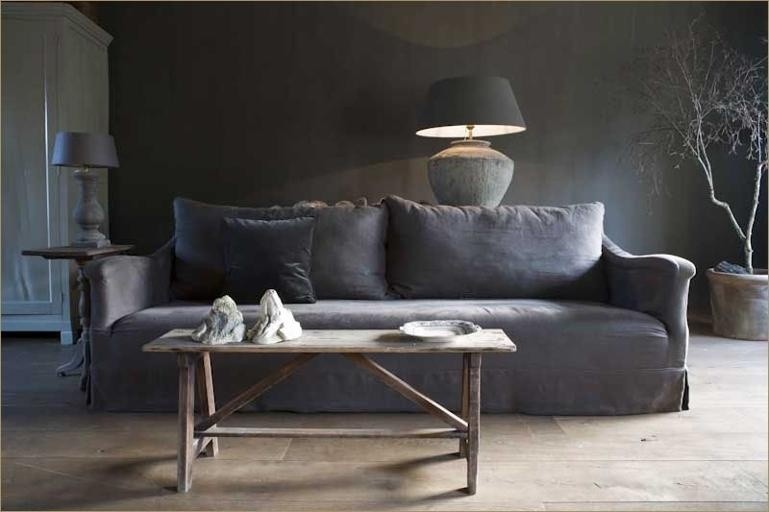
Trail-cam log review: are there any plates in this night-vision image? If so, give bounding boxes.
[399,318,483,344]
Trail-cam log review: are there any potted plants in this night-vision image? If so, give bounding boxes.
[604,10,769,341]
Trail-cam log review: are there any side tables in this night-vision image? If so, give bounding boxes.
[22,246,135,377]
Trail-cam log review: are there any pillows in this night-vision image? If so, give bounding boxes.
[218,216,318,305]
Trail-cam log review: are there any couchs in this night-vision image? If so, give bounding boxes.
[83,196,697,417]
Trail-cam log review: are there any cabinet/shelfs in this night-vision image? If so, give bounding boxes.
[0,2,113,347]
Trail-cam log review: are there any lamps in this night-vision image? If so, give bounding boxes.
[51,130,121,247]
[415,75,527,205]
